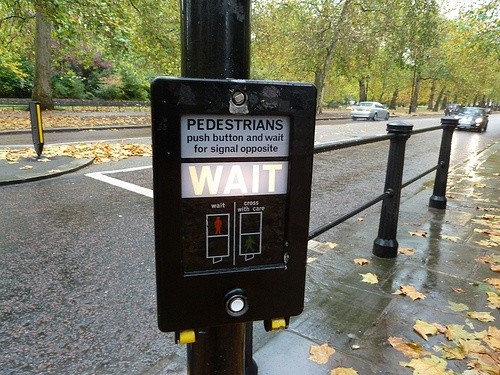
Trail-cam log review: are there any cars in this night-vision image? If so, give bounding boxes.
[351,101,389,121]
[445,103,492,133]
